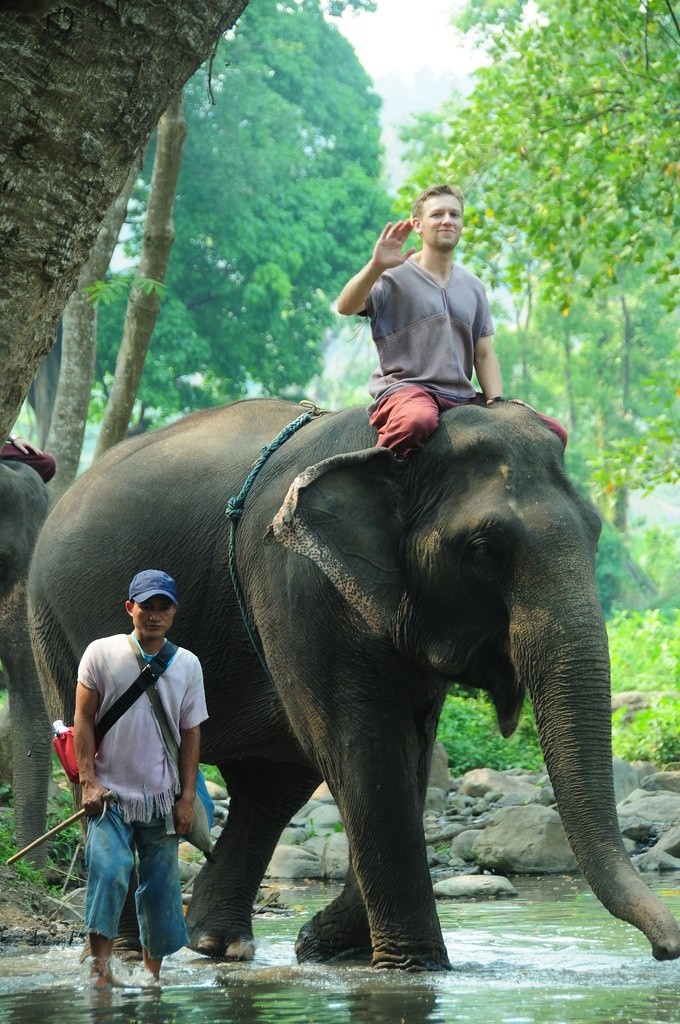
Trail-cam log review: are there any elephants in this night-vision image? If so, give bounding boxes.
[0,397,680,983]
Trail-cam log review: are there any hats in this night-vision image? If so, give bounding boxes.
[129,569,178,604]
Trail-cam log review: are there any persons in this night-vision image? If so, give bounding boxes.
[335,186,566,470]
[72,569,207,988]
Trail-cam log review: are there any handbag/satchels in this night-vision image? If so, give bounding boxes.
[182,763,231,863]
[28,728,97,784]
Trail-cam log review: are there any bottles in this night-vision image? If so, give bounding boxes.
[53,720,69,736]
